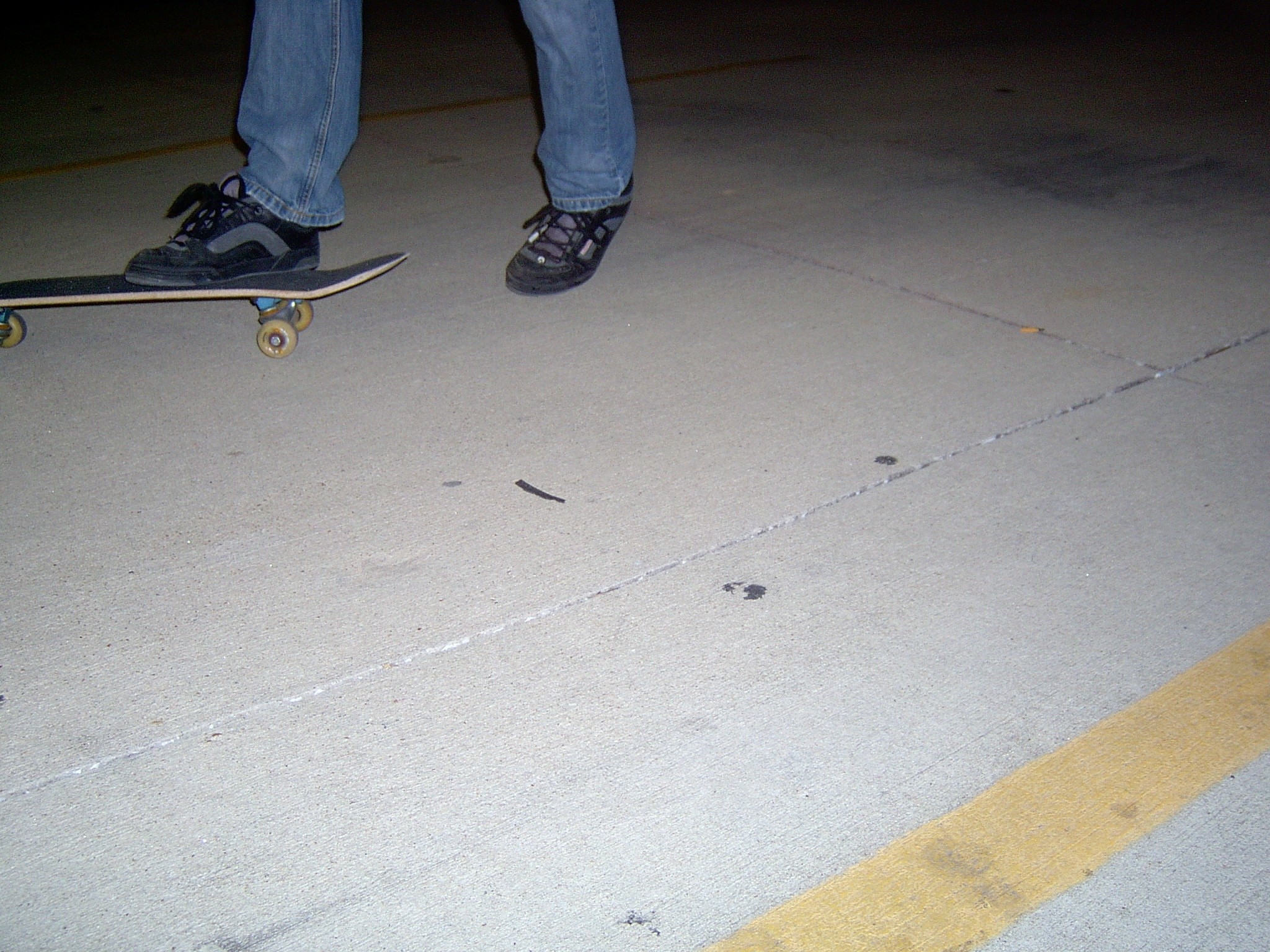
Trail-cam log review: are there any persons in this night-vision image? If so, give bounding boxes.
[123,0,638,297]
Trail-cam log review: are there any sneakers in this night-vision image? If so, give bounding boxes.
[506,172,635,296]
[125,171,320,287]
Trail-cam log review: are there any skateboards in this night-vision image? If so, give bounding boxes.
[0,249,413,358]
